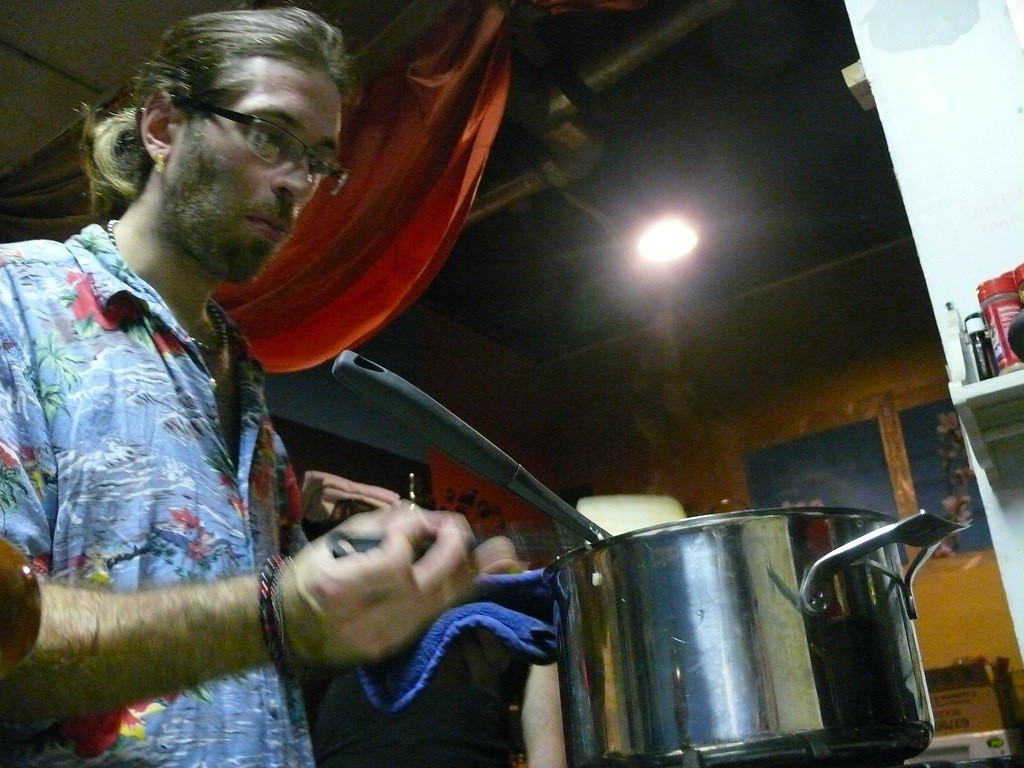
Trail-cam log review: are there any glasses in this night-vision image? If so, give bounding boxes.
[186,97,352,198]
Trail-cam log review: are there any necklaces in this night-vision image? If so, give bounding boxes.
[108,219,228,355]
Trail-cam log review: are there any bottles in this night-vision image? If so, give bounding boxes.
[965,262,1024,382]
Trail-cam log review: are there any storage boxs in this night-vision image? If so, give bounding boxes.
[924,656,1016,737]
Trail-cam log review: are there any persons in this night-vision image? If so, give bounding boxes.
[0,7,522,768]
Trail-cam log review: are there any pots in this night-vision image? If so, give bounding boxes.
[332,350,971,768]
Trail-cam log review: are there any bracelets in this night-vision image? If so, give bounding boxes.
[259,554,319,685]
